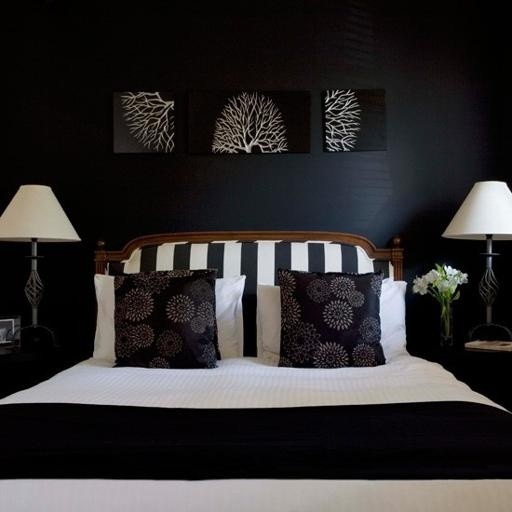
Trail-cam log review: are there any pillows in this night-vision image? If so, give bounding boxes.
[278,268,385,369]
[93,274,247,367]
[257,279,408,364]
[113,269,220,369]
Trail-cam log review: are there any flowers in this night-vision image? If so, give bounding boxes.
[411,263,468,340]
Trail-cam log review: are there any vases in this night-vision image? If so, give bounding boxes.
[438,334,454,349]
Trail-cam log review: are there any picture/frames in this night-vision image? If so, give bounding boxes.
[0,319,15,344]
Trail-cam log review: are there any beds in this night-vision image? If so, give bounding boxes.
[0,231,512,512]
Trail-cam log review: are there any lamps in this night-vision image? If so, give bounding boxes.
[0,185,81,344]
[441,181,512,351]
